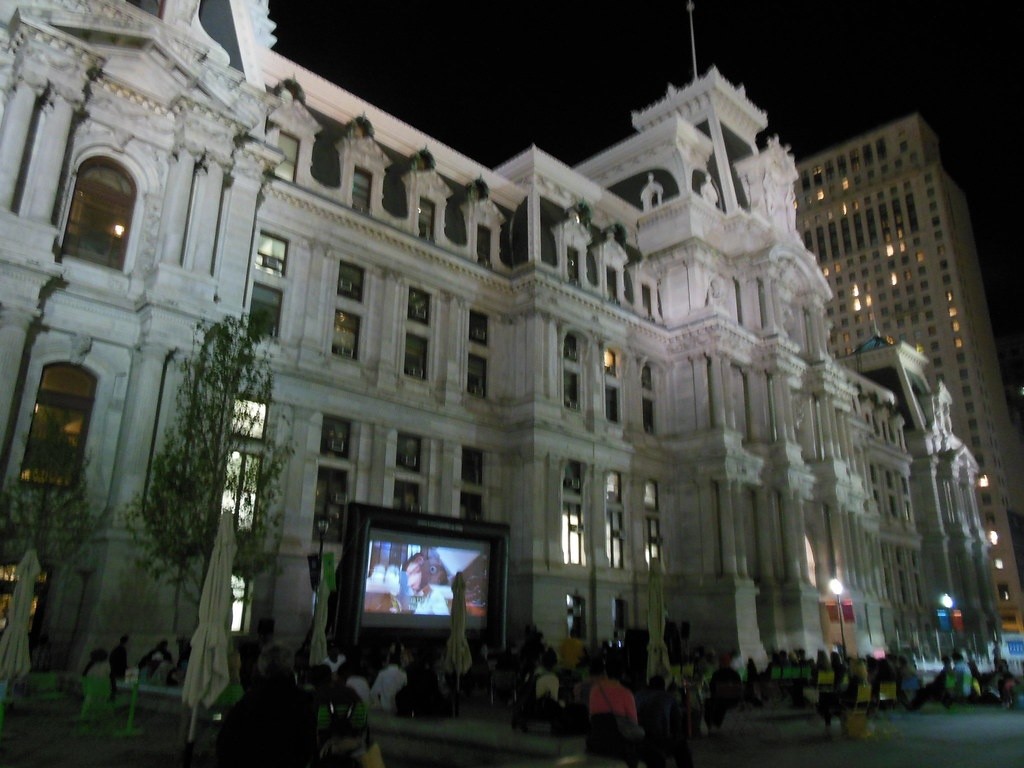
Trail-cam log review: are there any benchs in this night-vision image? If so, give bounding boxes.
[115,681,587,767]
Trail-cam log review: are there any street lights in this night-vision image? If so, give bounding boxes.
[827,577,848,660]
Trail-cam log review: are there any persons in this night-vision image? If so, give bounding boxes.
[83,616,1024,766]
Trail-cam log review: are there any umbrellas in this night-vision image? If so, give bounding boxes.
[178,512,234,750]
[2,549,41,694]
[440,571,473,692]
[308,579,333,667]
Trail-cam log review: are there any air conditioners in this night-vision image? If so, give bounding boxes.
[607,365,614,373]
[326,439,344,452]
[567,401,577,409]
[412,307,426,318]
[401,454,416,466]
[338,346,354,358]
[408,366,423,377]
[564,347,576,359]
[471,385,484,395]
[614,630,625,639]
[338,279,351,291]
[471,329,485,339]
[571,524,583,532]
[613,530,625,539]
[565,477,580,488]
[264,256,278,269]
[568,607,581,616]
[408,503,421,513]
[330,493,347,504]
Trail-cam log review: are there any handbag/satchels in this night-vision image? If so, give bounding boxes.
[349,725,385,768]
[615,717,646,742]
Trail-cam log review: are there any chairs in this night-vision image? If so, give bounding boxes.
[943,673,972,704]
[874,681,896,717]
[80,676,110,723]
[672,664,694,680]
[29,672,63,711]
[816,671,848,694]
[491,671,517,703]
[853,684,871,715]
[770,666,813,680]
[346,704,367,732]
[317,701,336,731]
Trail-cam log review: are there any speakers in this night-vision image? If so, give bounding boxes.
[257,618,275,638]
[680,621,691,641]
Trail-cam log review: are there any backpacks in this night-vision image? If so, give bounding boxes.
[320,697,358,767]
[517,665,554,707]
